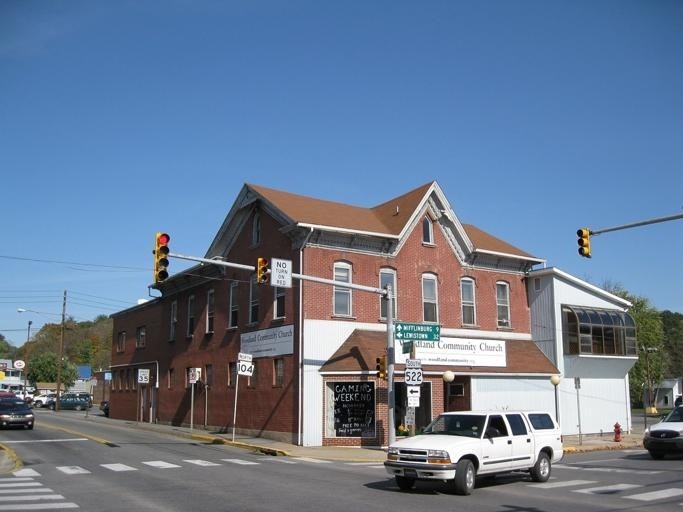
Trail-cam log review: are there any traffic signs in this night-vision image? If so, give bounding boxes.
[270,257,292,289]
[394,322,441,342]
[404,367,422,398]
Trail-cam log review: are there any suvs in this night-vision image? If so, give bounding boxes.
[383,410,564,496]
[643,405,683,459]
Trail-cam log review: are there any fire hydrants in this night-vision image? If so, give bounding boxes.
[612,422,623,442]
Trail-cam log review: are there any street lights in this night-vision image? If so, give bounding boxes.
[442,371,456,411]
[17,289,68,413]
[550,373,561,424]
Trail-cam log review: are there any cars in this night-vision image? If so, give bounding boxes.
[0,383,109,430]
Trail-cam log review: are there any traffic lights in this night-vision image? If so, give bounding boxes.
[376,354,387,380]
[153,232,171,282]
[575,228,591,257]
[257,257,269,284]
